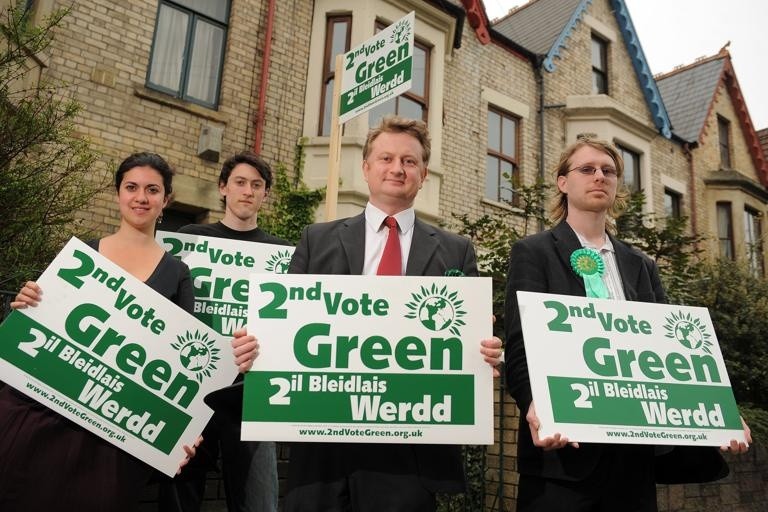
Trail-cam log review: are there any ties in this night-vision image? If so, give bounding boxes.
[376,216,402,276]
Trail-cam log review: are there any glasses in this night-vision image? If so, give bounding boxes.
[565,166,620,177]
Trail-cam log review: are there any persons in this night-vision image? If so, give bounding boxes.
[505,137,754,512]
[10,153,207,509]
[230,116,506,511]
[171,152,294,511]
